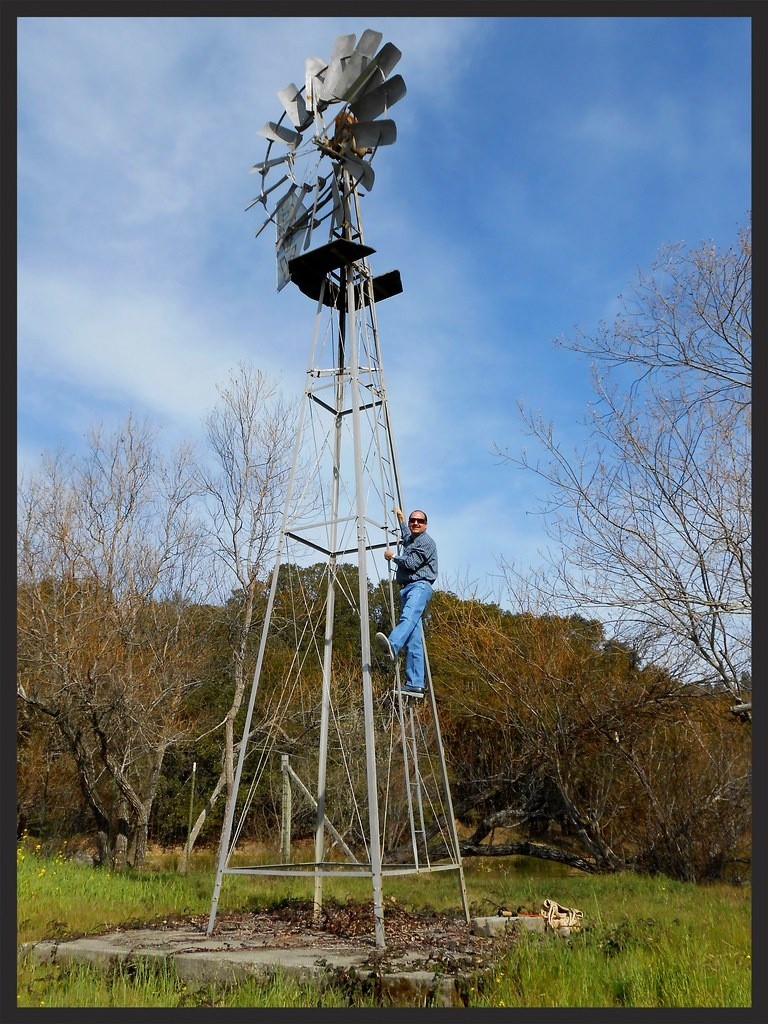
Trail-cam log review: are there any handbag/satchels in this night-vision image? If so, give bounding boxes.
[541,899,584,934]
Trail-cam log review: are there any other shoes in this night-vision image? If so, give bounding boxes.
[375,632,395,662]
[392,685,424,698]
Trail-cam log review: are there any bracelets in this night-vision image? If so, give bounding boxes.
[392,554,396,561]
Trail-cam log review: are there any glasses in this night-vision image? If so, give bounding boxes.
[408,518,426,524]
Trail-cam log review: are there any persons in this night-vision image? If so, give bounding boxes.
[326,112,374,156]
[376,508,438,698]
